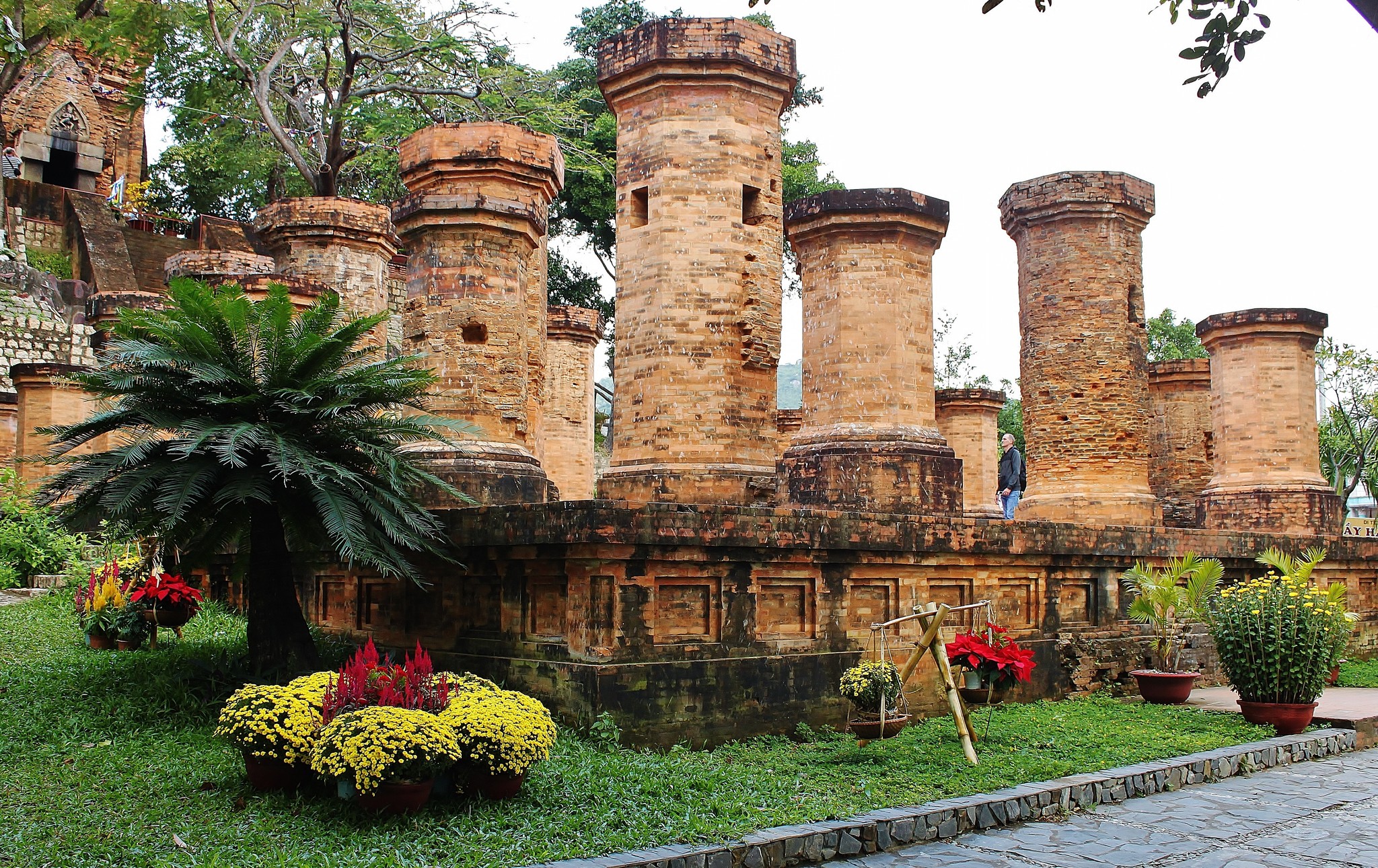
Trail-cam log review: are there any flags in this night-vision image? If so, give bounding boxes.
[106,174,125,206]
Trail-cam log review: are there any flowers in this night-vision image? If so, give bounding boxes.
[944,622,1037,689]
[108,180,158,221]
[839,659,904,714]
[73,553,206,642]
[213,637,561,797]
[1206,570,1356,705]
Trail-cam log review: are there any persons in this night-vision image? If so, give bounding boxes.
[1,147,23,178]
[994,433,1026,520]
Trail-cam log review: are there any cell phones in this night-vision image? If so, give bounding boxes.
[997,491,1004,495]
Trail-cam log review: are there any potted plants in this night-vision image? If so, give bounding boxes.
[157,211,185,238]
[1254,545,1363,685]
[1119,549,1225,704]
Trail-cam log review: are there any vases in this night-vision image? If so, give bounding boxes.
[362,782,432,815]
[126,219,154,232]
[958,688,1010,704]
[118,638,141,651]
[468,769,526,801]
[143,610,193,627]
[244,753,315,793]
[849,713,908,739]
[85,634,114,650]
[962,670,983,689]
[1237,700,1319,735]
[338,778,373,801]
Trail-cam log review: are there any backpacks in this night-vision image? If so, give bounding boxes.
[1012,447,1027,490]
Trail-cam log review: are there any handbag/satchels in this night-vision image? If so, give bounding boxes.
[13,169,22,176]
